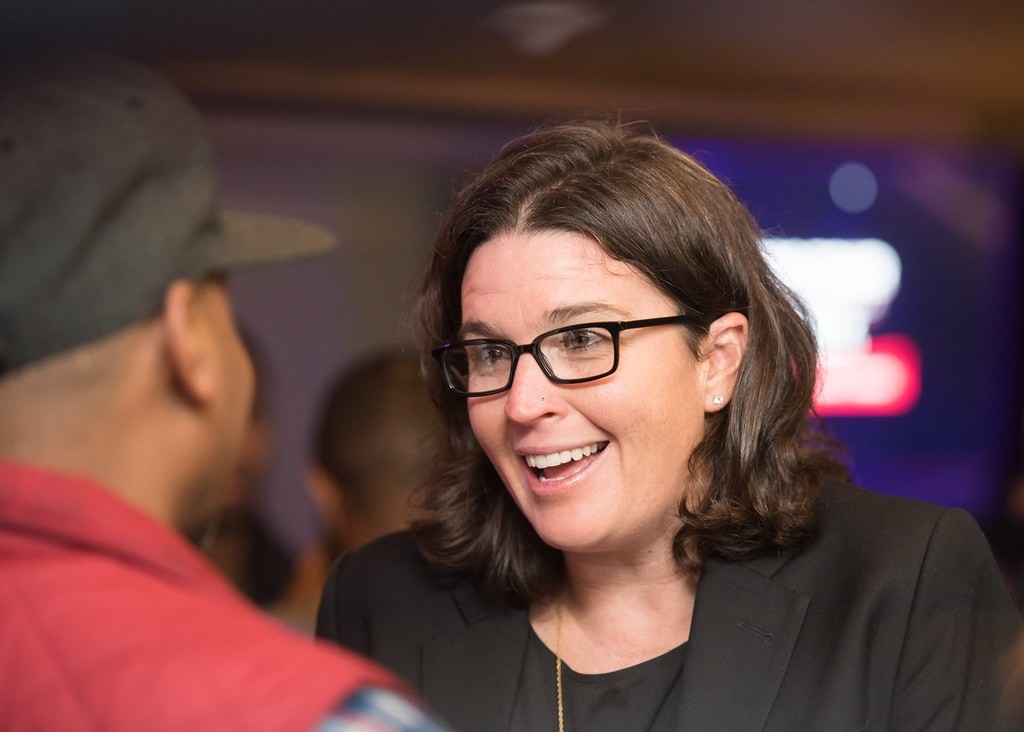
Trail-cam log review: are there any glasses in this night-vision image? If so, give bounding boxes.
[432,312,722,397]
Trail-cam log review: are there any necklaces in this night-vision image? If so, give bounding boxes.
[555,595,564,732]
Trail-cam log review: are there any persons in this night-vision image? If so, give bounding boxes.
[176,322,291,610]
[315,124,1023,731]
[265,348,470,637]
[0,58,453,731]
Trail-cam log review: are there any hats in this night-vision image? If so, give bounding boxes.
[1,67,339,372]
